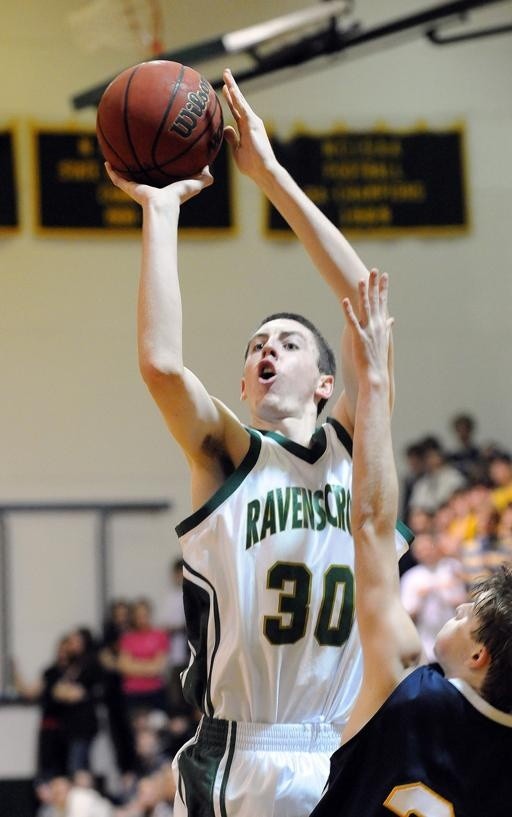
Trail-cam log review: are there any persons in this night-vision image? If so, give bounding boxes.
[34,708,202,817]
[104,69,418,816]
[35,597,174,787]
[159,556,188,673]
[401,413,512,666]
[315,266,512,816]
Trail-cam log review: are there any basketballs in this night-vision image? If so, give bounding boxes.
[97,59,224,187]
